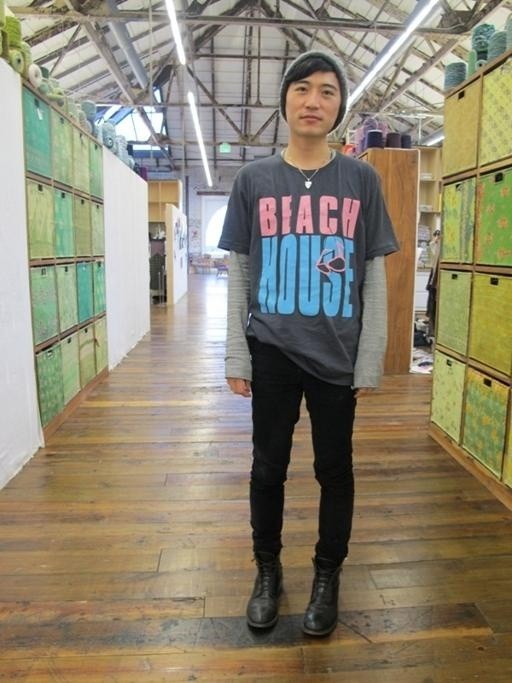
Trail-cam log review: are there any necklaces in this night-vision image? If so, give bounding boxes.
[288,156,325,190]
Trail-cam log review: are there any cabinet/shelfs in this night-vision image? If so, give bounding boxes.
[21,75,109,442]
[358,146,420,377]
[146,179,182,305]
[427,56,510,514]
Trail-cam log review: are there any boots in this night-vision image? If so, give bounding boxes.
[301,552,342,636]
[243,541,284,632]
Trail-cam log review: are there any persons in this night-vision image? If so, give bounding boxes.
[211,48,401,641]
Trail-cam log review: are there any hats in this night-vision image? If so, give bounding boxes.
[279,50,348,135]
